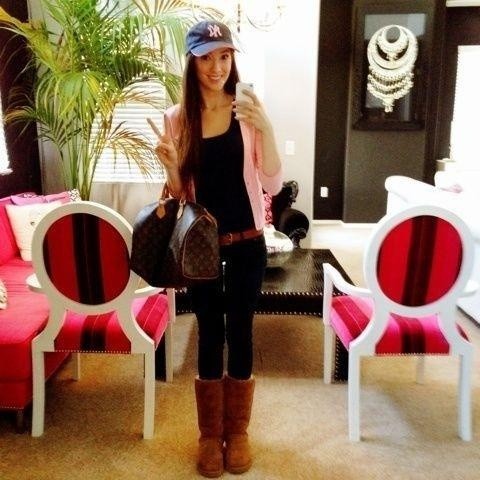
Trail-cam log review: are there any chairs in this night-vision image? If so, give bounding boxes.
[313,207,474,444]
[25,200,177,440]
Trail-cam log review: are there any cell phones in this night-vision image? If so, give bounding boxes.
[235,82,254,120]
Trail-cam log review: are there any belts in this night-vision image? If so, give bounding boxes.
[219,228,264,247]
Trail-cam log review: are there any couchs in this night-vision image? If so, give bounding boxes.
[0,187,94,433]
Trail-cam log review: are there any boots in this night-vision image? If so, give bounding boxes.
[195,375,256,477]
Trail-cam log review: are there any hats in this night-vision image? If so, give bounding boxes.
[185,20,241,58]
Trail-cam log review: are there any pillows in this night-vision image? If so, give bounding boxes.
[8,192,84,260]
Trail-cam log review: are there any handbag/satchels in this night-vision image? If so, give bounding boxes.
[128,196,218,287]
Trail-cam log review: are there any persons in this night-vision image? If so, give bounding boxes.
[146,20,284,478]
